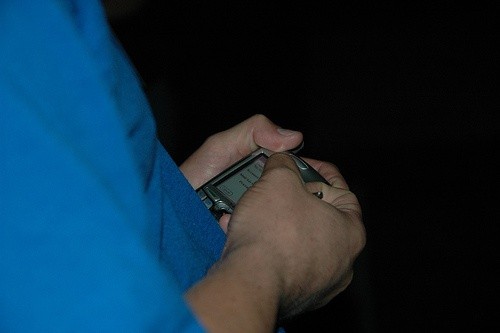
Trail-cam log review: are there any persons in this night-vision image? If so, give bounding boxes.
[0,0,365,333]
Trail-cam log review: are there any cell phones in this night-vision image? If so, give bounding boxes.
[196,148,333,221]
